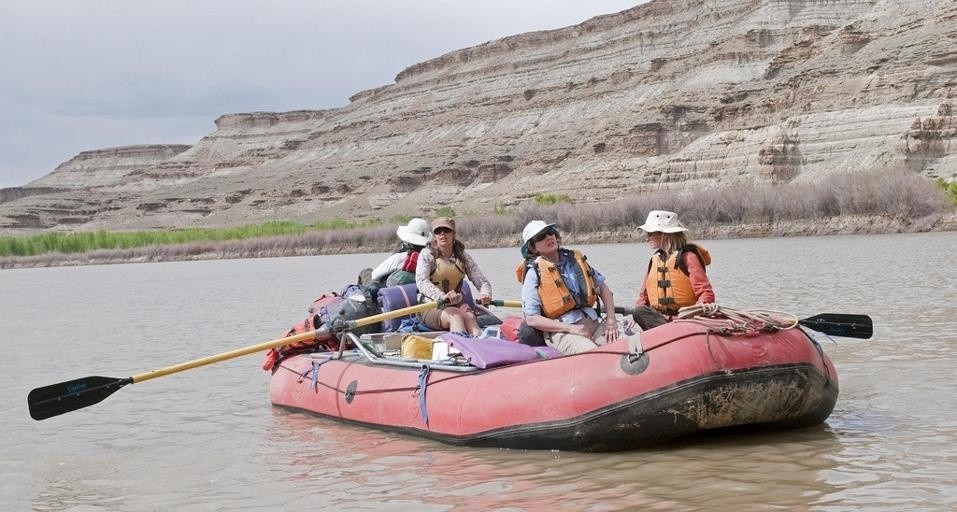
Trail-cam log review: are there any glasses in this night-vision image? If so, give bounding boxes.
[531,229,555,242]
[433,227,452,234]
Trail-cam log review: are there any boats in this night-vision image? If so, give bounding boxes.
[267,291,841,452]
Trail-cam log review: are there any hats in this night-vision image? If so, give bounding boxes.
[520,219,557,258]
[635,209,688,234]
[431,217,456,231]
[396,217,434,247]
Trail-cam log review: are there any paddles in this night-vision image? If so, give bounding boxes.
[476,299,872,338]
[28,293,463,420]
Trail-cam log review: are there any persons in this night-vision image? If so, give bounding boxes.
[357,218,433,291]
[625,210,715,332]
[520,218,629,356]
[415,218,492,337]
